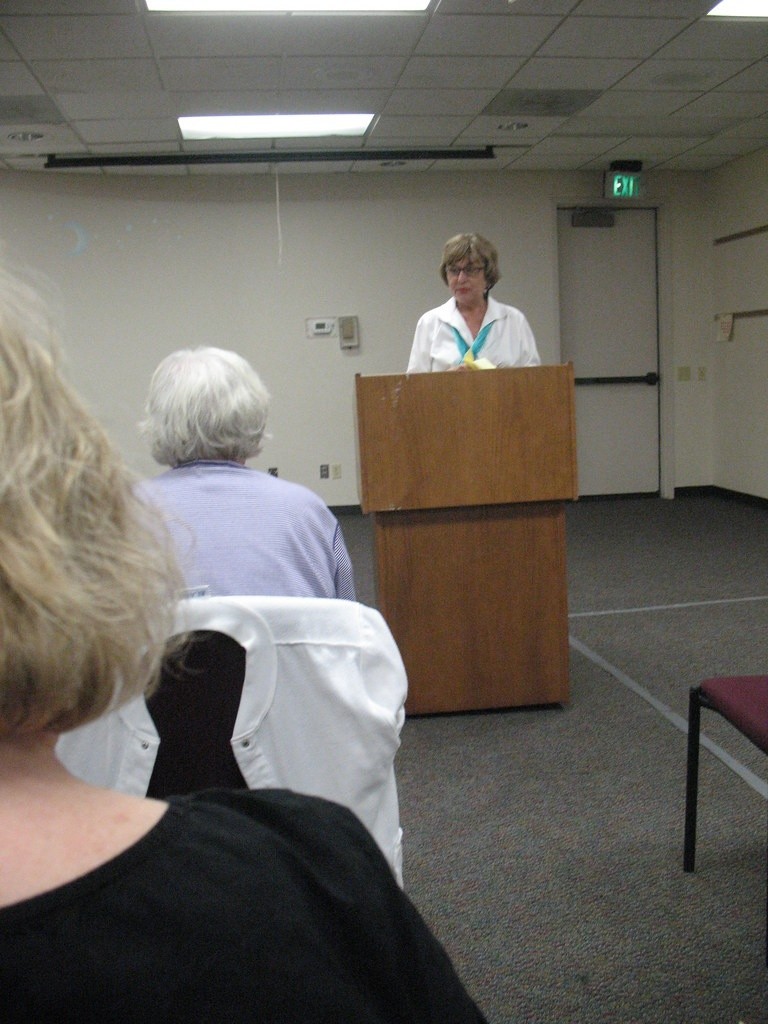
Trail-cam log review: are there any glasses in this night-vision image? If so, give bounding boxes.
[447,265,485,277]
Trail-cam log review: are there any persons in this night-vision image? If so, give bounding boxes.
[407,233,543,374]
[0,261,497,1024]
[129,348,355,600]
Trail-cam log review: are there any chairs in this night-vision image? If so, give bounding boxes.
[53,595,409,889]
[683,675,768,874]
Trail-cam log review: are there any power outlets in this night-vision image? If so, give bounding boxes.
[331,463,342,480]
[320,464,329,478]
